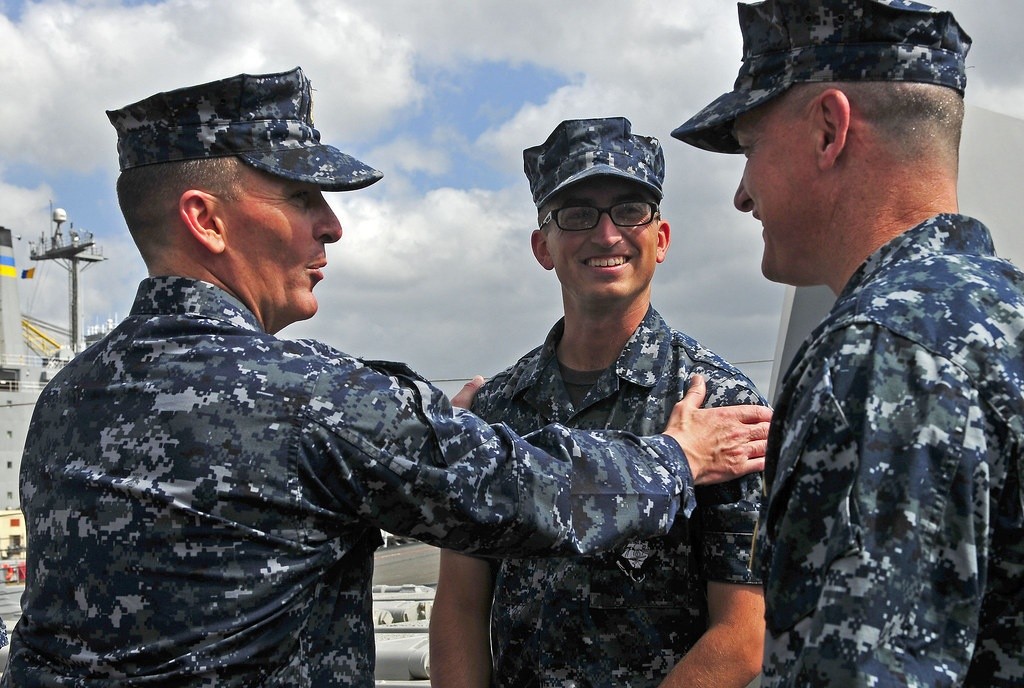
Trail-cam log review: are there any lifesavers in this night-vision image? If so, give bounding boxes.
[0,564,14,580]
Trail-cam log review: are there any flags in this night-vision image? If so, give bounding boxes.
[21,268,34,279]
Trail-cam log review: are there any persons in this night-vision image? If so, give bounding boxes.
[0,67,773,688]
[672,1,1024,686]
[427,115,772,688]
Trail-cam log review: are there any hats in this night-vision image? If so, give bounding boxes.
[105,66,384,192]
[671,0,973,154]
[523,118,665,206]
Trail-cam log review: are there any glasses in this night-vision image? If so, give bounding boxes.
[539,200,661,232]
